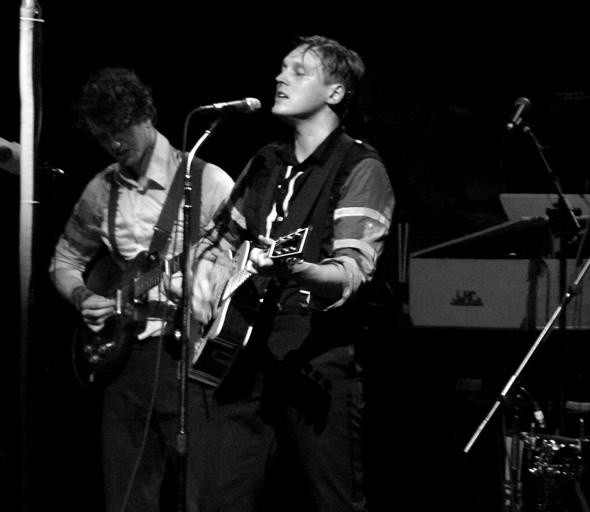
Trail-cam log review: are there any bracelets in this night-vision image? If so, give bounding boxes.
[74,287,84,312]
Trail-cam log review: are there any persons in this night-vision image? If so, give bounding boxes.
[47,68,236,509]
[185,34,397,508]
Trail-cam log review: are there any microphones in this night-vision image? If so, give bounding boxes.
[204,97,263,115]
[506,97,532,128]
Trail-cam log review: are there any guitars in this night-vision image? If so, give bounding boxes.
[186,227,309,390]
[70,248,190,385]
